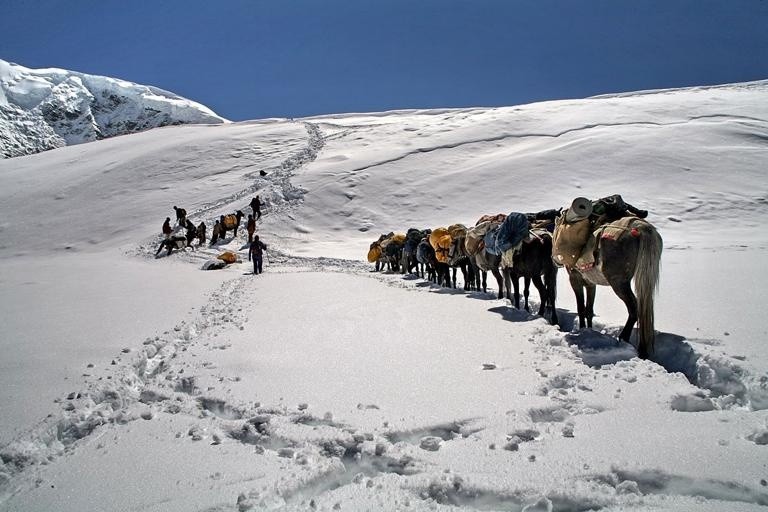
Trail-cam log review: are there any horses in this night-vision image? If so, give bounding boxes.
[219,210,244,239]
[368,206,565,326]
[156,229,198,256]
[566,211,664,361]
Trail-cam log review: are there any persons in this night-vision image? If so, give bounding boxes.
[184,219,196,238]
[248,235,267,274]
[163,217,174,249]
[211,219,223,244]
[173,206,186,227]
[196,221,207,242]
[249,195,265,220]
[245,214,255,244]
